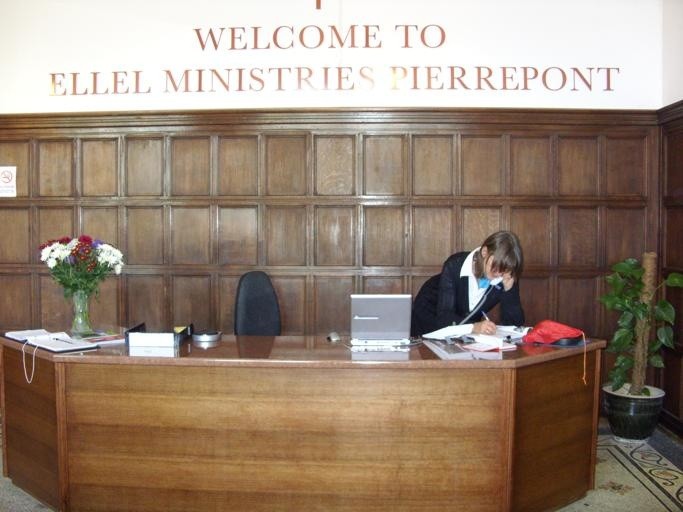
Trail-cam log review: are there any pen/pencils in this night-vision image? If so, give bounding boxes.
[481,310,490,321]
[53,338,74,344]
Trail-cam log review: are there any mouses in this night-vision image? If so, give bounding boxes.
[326,331,341,341]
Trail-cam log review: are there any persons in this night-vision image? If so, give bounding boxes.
[410,230,525,337]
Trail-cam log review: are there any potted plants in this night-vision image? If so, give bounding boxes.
[597,250,682,451]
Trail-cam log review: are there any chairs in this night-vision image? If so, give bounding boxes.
[232,270,281,339]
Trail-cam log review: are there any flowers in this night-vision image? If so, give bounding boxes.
[37,233,127,326]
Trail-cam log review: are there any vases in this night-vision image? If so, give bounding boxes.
[69,289,92,335]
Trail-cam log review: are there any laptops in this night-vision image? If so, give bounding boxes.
[348,294,423,346]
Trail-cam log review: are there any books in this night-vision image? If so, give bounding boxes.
[71,331,125,347]
[1,329,102,354]
[420,323,534,352]
